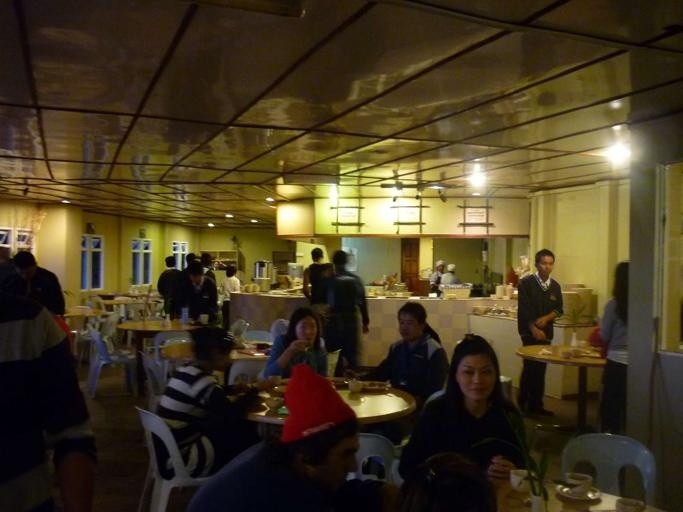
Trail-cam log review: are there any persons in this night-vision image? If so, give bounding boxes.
[429,260,462,297]
[344,302,449,445]
[263,307,327,377]
[186,364,403,512]
[303,247,369,376]
[598,261,628,437]
[398,334,537,486]
[154,328,264,478]
[157,253,227,325]
[219,266,241,331]
[403,453,498,512]
[517,248,555,418]
[0,244,97,512]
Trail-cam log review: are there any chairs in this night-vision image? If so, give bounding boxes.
[55,292,664,512]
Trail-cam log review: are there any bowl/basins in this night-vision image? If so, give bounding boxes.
[98,294,118,301]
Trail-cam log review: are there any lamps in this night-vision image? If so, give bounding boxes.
[393,182,448,202]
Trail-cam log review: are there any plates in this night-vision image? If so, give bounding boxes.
[556,483,601,500]
[271,384,287,393]
[360,380,393,393]
[325,376,353,388]
[558,330,595,359]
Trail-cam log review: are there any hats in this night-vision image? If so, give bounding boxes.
[278,364,355,443]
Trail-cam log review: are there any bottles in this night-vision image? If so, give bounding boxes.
[181,307,189,323]
[162,313,170,328]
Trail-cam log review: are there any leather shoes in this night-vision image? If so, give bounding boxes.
[533,407,553,416]
[518,400,528,414]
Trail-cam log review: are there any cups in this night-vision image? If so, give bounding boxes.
[264,397,284,413]
[566,472,591,495]
[495,285,513,299]
[368,288,376,294]
[348,378,363,393]
[288,280,294,289]
[199,314,208,324]
[472,305,517,319]
[613,497,646,512]
[510,468,537,490]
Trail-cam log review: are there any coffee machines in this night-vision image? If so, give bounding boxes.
[254,260,274,289]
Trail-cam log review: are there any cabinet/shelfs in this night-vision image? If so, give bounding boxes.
[200,243,239,271]
